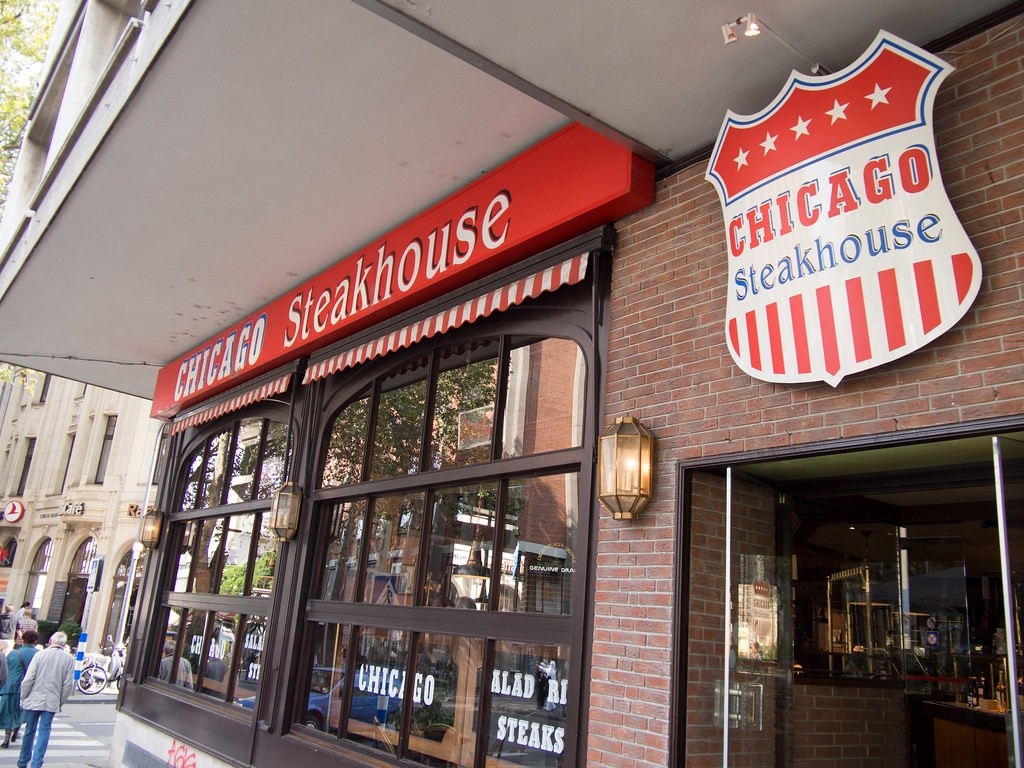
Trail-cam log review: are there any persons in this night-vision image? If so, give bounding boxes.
[104,635,114,656]
[244,651,256,679]
[537,660,558,712]
[203,644,229,699]
[0,602,41,748]
[338,644,368,698]
[156,641,193,693]
[17,630,76,768]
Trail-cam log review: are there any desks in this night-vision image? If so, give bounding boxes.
[922,698,1010,768]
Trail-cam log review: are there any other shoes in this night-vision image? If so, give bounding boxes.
[1,742,8,748]
[11,729,18,742]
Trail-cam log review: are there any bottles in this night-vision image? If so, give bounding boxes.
[966,676,987,707]
[996,671,1007,713]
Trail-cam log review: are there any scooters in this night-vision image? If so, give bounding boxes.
[85,635,130,692]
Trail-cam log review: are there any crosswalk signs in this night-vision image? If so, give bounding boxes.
[369,574,408,606]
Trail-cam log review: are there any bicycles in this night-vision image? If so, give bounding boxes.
[77,643,107,696]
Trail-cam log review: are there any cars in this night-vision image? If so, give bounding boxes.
[236,666,421,736]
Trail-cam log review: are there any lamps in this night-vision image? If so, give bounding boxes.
[597,416,654,521]
[268,480,303,542]
[139,504,164,551]
